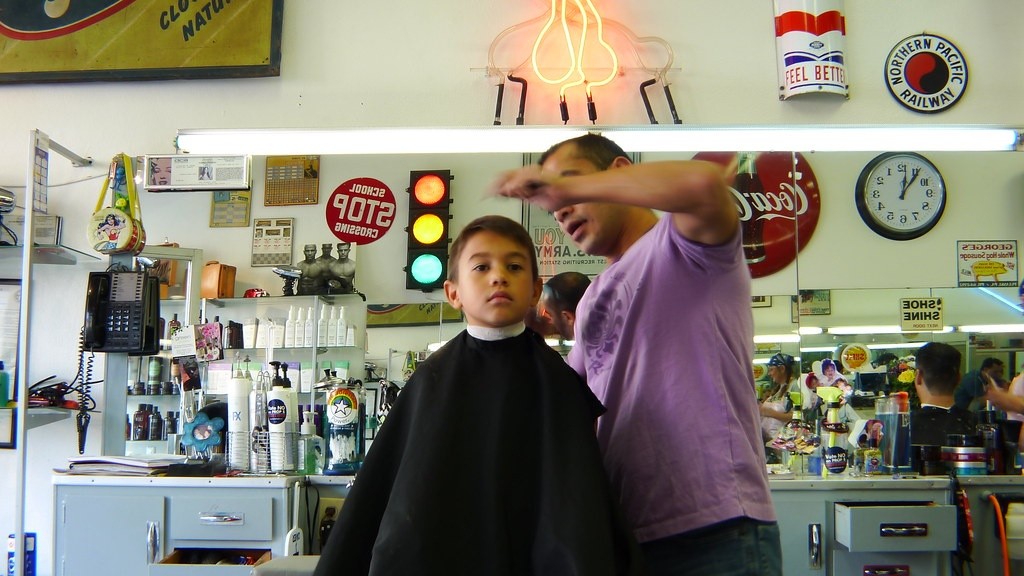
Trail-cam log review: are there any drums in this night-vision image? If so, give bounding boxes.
[88,206,139,254]
[132,217,146,256]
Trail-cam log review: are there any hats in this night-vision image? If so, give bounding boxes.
[765,352,795,367]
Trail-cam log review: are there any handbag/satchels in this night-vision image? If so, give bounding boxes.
[87,153,139,254]
[134,187,146,256]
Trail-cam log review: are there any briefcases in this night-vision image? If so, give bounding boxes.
[200,260,237,298]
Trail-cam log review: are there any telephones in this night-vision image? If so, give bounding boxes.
[84,270,159,356]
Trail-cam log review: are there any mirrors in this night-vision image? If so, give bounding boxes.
[100,244,203,456]
[365,303,577,456]
[749,289,1024,476]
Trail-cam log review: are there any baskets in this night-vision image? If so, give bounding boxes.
[225,431,301,477]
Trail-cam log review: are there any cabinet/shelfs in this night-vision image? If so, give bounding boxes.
[201,294,367,467]
[0,244,101,416]
[124,299,188,456]
[50,474,357,576]
[769,476,957,575]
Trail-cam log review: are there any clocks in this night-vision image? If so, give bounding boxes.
[854,152,947,241]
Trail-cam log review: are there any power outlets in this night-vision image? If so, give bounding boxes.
[318,497,345,540]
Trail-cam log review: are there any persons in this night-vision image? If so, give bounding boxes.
[1007,364,1024,421]
[893,342,1006,476]
[986,279,1024,475]
[491,133,782,576]
[878,352,899,366]
[955,358,1011,413]
[314,215,645,576]
[805,373,824,408]
[200,167,211,180]
[536,272,591,341]
[760,353,803,464]
[822,360,847,404]
[151,159,171,186]
[867,423,884,448]
[204,430,211,439]
[193,428,203,439]
[297,242,356,294]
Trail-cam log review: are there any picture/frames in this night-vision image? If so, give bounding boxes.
[367,303,463,328]
[365,389,377,440]
[0,1,284,84]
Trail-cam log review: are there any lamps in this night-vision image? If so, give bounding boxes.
[800,324,1024,335]
[175,125,1024,159]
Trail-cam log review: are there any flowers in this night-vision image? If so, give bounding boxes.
[889,355,917,384]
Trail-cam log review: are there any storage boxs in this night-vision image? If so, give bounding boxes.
[7,533,36,576]
[288,361,350,393]
[207,362,262,390]
